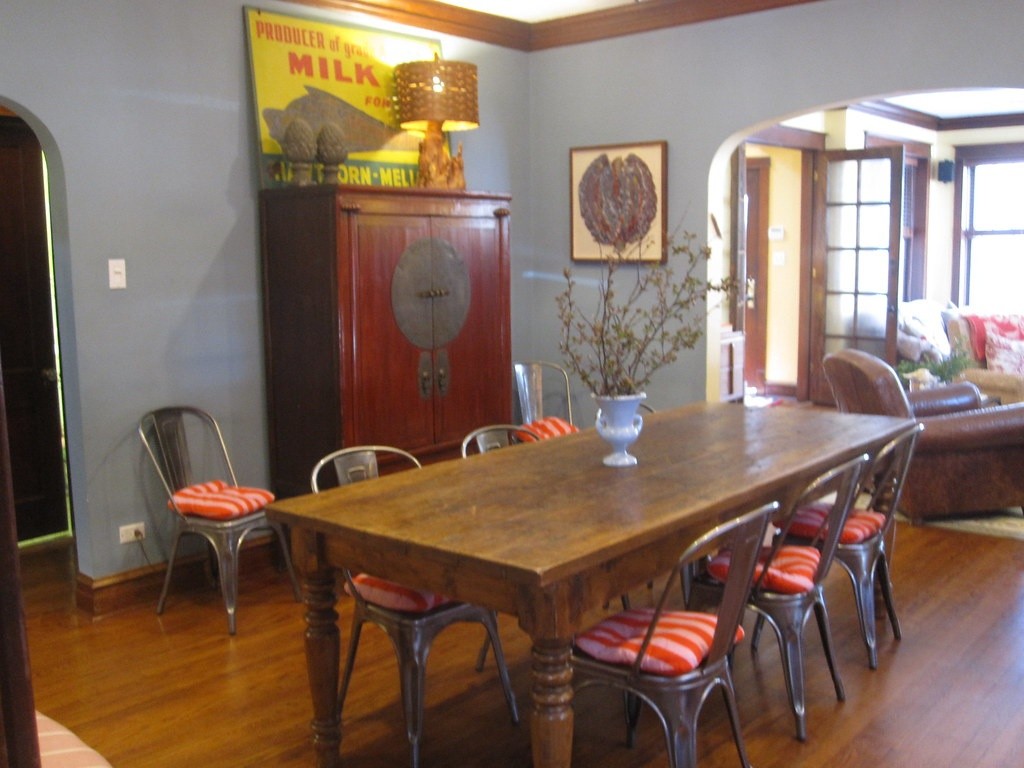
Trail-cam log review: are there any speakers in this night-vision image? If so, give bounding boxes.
[937,159,954,184]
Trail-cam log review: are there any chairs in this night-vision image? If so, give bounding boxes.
[569,500,779,768]
[749,423,924,673]
[511,360,580,444]
[309,445,519,768]
[461,424,630,611]
[137,407,302,635]
[689,454,870,741]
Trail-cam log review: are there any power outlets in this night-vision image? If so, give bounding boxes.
[120,523,144,544]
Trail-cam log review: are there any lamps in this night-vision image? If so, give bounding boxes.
[393,53,479,191]
[937,158,956,183]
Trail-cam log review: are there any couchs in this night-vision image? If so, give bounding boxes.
[940,306,1024,404]
[843,299,961,364]
[823,349,1024,527]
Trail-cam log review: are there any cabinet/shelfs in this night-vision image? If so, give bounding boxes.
[257,184,512,566]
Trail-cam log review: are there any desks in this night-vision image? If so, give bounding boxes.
[271,401,922,768]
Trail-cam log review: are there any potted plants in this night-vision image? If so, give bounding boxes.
[555,220,739,467]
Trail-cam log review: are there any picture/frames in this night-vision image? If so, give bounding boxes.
[567,140,666,263]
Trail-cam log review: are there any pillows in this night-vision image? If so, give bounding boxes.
[964,313,1024,360]
[985,332,1024,376]
